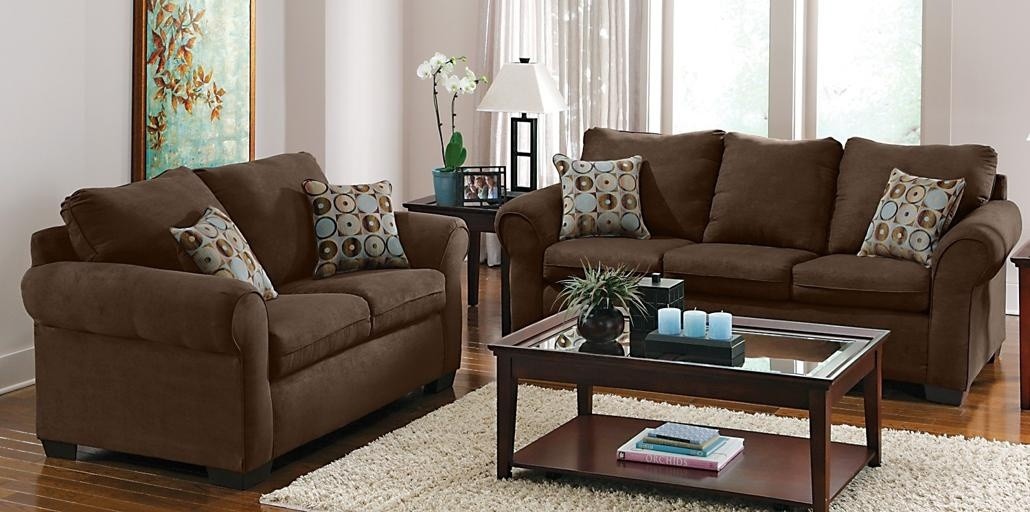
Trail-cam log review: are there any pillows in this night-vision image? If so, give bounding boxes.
[168,208,280,302]
[548,153,650,240]
[298,175,407,279]
[858,168,965,268]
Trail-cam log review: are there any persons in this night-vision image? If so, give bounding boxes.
[464,175,498,200]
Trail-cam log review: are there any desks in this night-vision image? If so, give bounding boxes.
[489,294,895,510]
[1008,242,1030,418]
[402,189,524,308]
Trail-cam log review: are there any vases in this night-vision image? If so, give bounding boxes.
[430,167,463,207]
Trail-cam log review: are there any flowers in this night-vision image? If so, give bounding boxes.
[417,48,489,170]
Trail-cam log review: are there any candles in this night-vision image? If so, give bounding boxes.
[654,299,681,336]
[706,311,733,340]
[681,309,708,340]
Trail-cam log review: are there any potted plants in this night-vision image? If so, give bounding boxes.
[553,258,650,344]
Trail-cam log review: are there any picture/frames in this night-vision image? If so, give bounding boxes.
[457,165,508,209]
[130,0,257,185]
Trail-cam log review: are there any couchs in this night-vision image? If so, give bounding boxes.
[17,149,476,493]
[495,122,1025,412]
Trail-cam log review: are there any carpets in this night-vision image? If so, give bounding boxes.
[253,370,1027,511]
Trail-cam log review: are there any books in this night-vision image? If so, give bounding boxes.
[617,422,743,472]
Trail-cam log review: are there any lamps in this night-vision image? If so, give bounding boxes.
[476,55,569,194]
